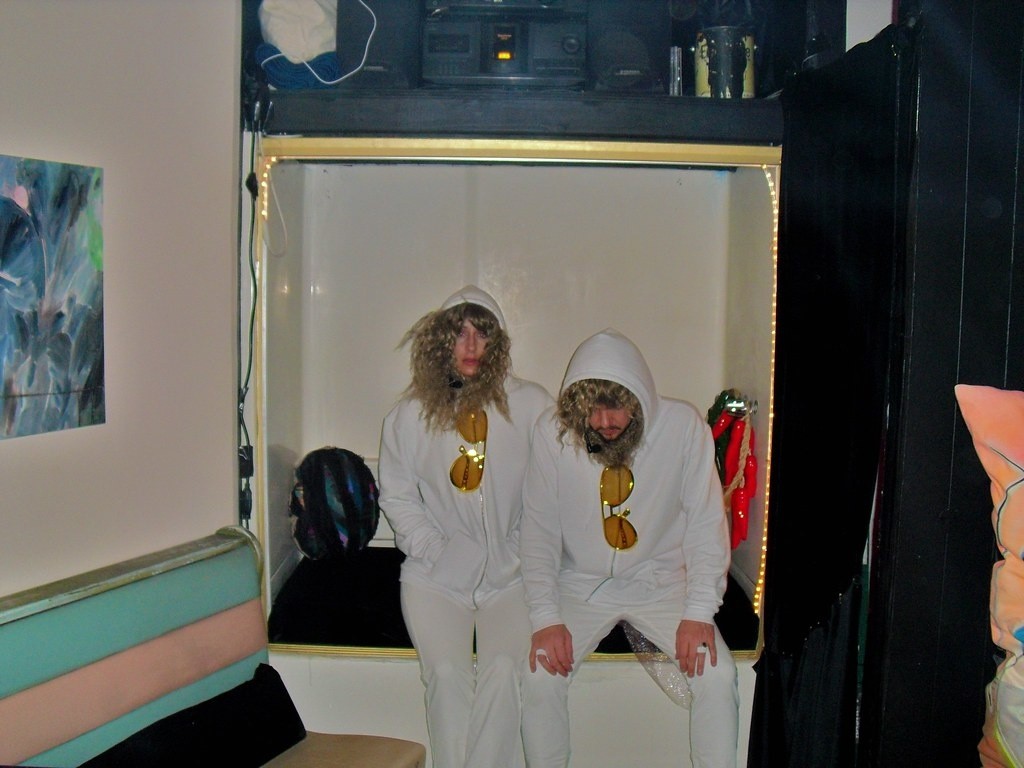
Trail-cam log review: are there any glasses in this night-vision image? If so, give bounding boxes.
[600,460,638,550]
[449,403,488,493]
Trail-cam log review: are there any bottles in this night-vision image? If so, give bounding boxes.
[668,46,684,97]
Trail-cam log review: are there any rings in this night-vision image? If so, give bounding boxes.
[696,646,706,653]
[536,649,547,658]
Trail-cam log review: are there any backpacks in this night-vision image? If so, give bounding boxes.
[289,446,380,573]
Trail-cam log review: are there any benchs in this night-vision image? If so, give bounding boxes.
[0,526,427,768]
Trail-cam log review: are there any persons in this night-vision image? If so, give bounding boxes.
[378,285,557,768]
[520,328,739,768]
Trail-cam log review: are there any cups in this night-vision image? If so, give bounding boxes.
[702,26,746,99]
